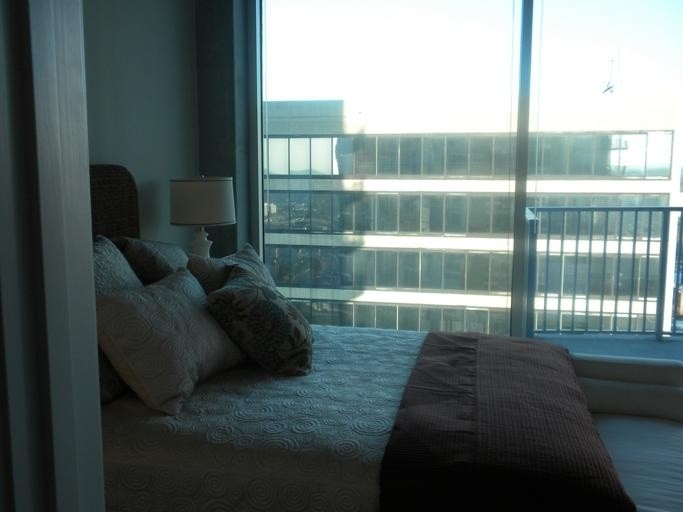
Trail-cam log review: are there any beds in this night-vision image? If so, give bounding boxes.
[91,164,682,511]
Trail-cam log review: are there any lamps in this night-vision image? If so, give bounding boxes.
[163,174,238,258]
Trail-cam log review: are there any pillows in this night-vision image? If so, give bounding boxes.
[112,233,193,286]
[93,265,239,416]
[92,234,148,409]
[202,261,315,380]
[187,242,279,294]
